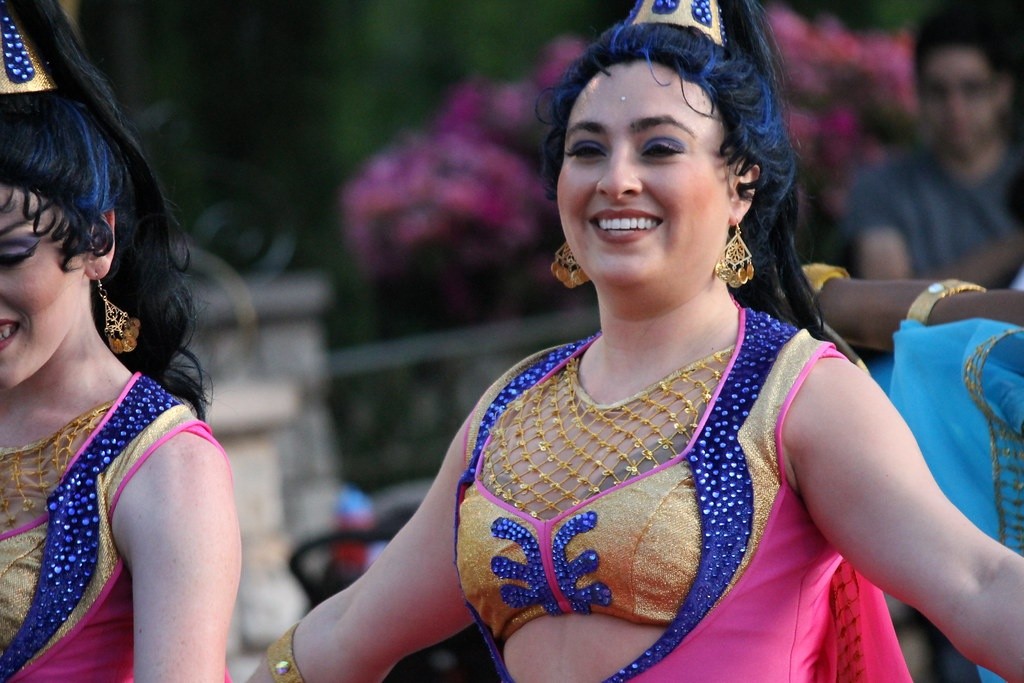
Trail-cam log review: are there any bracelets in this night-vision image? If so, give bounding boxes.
[799,261,852,295]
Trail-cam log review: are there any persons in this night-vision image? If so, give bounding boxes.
[244,0,1024,683]
[799,231,1024,683]
[0,0,242,683]
[842,0,1024,290]
